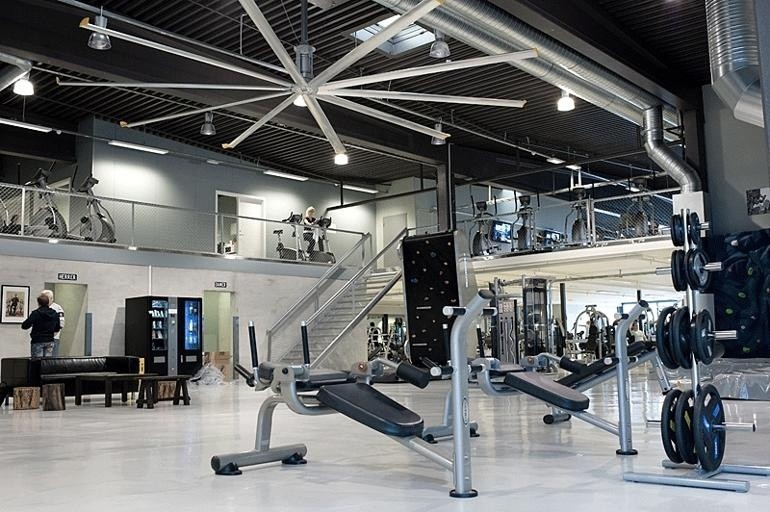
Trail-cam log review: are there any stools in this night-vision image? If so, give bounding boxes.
[1,356,192,411]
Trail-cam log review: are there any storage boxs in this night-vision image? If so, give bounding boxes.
[206,351,233,382]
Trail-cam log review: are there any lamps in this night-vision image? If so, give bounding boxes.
[432,124,446,146]
[557,89,576,112]
[87,7,110,50]
[14,63,35,97]
[201,111,217,135]
[428,28,451,58]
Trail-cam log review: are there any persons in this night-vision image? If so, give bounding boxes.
[8,294,19,314]
[20,294,60,357]
[630,322,649,344]
[366,322,379,348]
[302,206,318,258]
[40,290,65,357]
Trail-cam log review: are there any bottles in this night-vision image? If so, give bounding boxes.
[189,302,194,315]
[189,333,193,344]
[189,319,193,331]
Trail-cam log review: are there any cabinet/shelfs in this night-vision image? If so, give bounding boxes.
[125,297,203,377]
